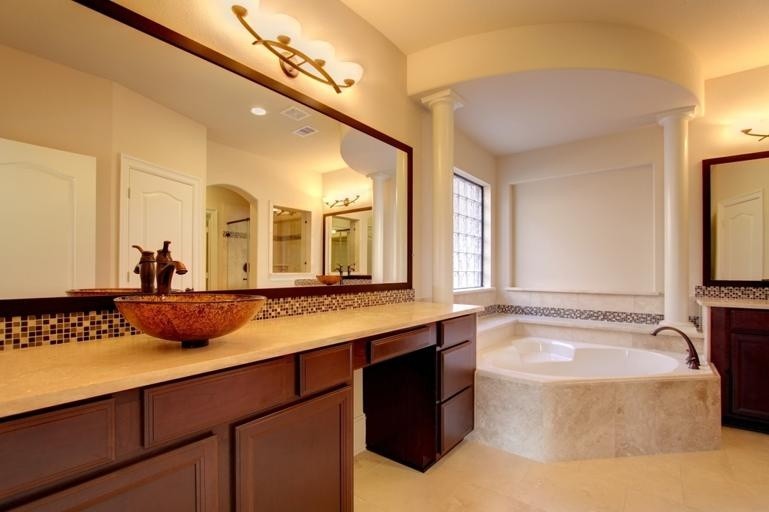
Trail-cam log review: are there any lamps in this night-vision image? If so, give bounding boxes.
[227,2,363,95]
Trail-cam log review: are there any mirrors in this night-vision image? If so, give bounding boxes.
[0,0,413,318]
[701,150,769,288]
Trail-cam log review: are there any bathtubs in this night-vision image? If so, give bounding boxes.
[470,331,713,383]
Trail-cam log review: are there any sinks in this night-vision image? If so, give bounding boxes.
[65,287,195,296]
[315,274,341,285]
[113,293,267,348]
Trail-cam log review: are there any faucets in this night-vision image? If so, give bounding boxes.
[334,262,343,285]
[131,244,155,293]
[155,240,189,294]
[348,264,355,276]
[650,325,701,367]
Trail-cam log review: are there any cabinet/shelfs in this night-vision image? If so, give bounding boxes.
[0,343,353,512]
[352,313,475,474]
[711,306,769,435]
